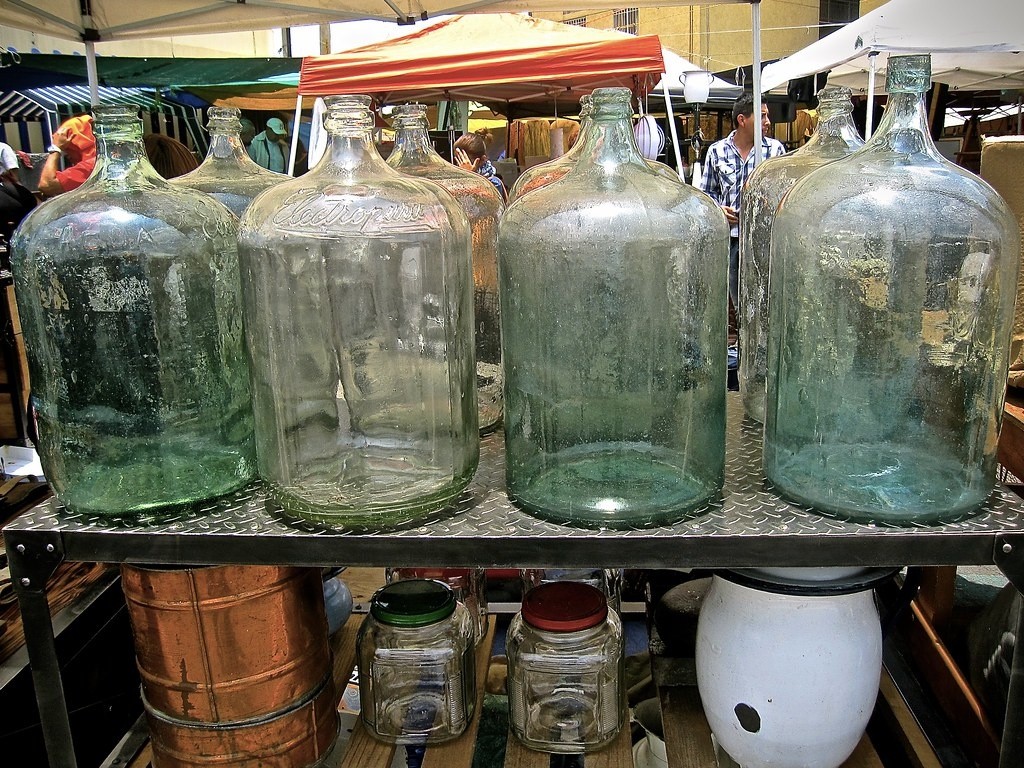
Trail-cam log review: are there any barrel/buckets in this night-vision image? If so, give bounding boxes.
[121,562,340,768]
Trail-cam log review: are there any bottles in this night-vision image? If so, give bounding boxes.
[9,52,1020,754]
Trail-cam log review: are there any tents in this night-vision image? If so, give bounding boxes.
[263,5,667,179]
[575,20,743,184]
[760,0,1023,146]
[0,0,763,190]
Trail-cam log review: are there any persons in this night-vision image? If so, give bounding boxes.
[287,135,309,177]
[247,117,289,175]
[0,141,38,358]
[142,133,198,182]
[36,113,96,195]
[701,86,786,372]
[450,126,510,203]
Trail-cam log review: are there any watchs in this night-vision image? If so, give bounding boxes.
[47,144,62,157]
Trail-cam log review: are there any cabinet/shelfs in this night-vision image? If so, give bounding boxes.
[1,387,1024,768]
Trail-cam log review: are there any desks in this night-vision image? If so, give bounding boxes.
[997,391,1024,487]
